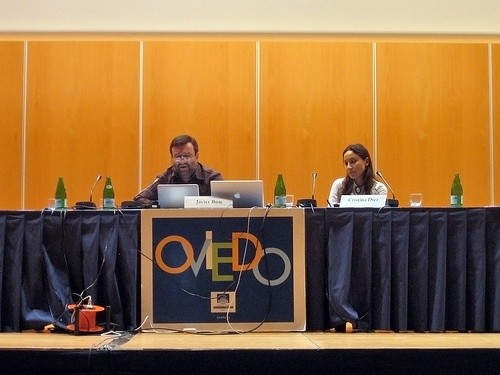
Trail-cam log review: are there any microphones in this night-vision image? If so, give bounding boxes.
[297,172,317,207]
[76,176,102,209]
[377,171,399,207]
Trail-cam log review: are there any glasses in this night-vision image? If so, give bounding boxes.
[171,154,197,164]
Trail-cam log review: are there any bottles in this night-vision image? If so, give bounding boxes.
[102,176,115,210]
[451,172,466,209]
[53,176,68,211]
[274,174,286,208]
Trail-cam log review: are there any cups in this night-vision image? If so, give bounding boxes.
[99,199,103,209]
[48,198,56,211]
[409,193,422,207]
[286,195,294,208]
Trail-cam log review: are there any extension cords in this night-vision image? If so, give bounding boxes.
[184,327,196,333]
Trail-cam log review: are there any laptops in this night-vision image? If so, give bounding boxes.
[210,180,264,208]
[158,184,199,208]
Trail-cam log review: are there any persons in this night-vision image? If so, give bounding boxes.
[132,134,226,208]
[326,144,388,207]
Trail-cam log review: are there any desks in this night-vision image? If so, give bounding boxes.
[0,207,500,332]
